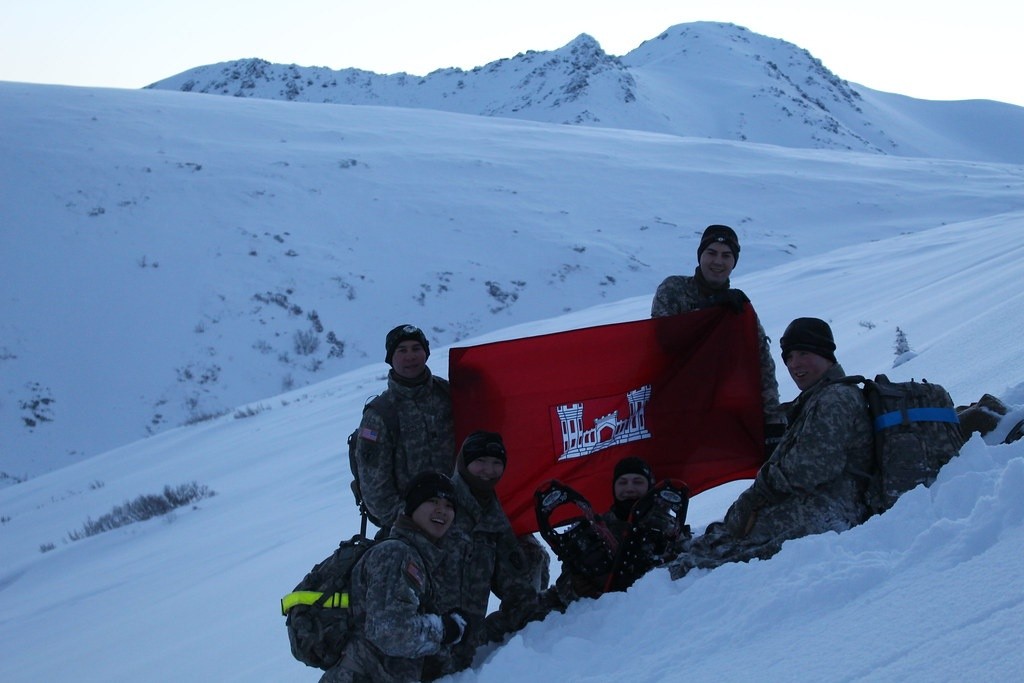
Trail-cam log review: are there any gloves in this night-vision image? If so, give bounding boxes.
[714,289,750,313]
[442,609,471,645]
[724,487,768,538]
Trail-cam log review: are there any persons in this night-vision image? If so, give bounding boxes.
[541,457,689,616]
[651,225,788,452]
[439,430,539,675]
[669,318,874,578]
[355,324,551,593]
[320,470,467,683]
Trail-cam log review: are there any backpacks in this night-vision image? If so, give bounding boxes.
[834,374,963,509]
[347,395,400,536]
[281,534,411,671]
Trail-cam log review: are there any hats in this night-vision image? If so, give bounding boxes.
[697,225,740,262]
[780,318,836,351]
[614,457,650,483]
[385,325,430,367]
[463,431,506,470]
[405,471,457,516]
